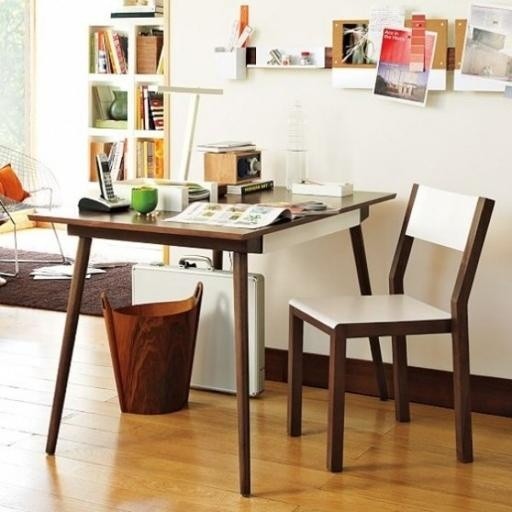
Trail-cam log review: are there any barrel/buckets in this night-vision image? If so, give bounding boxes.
[99,280,203,415]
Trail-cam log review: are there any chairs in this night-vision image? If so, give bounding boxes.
[0,144,72,279]
[287,183,495,473]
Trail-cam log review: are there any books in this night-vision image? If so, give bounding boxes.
[227,179,275,196]
[92,29,165,178]
[161,200,341,231]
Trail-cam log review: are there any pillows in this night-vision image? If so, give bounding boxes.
[0,163,30,202]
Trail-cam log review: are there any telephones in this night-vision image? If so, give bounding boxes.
[77,151,131,214]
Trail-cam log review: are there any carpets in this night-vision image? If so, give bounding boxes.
[0,245,138,316]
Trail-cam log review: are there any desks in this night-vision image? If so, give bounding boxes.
[26,186,397,497]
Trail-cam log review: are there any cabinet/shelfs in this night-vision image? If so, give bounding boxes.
[86,0,170,183]
